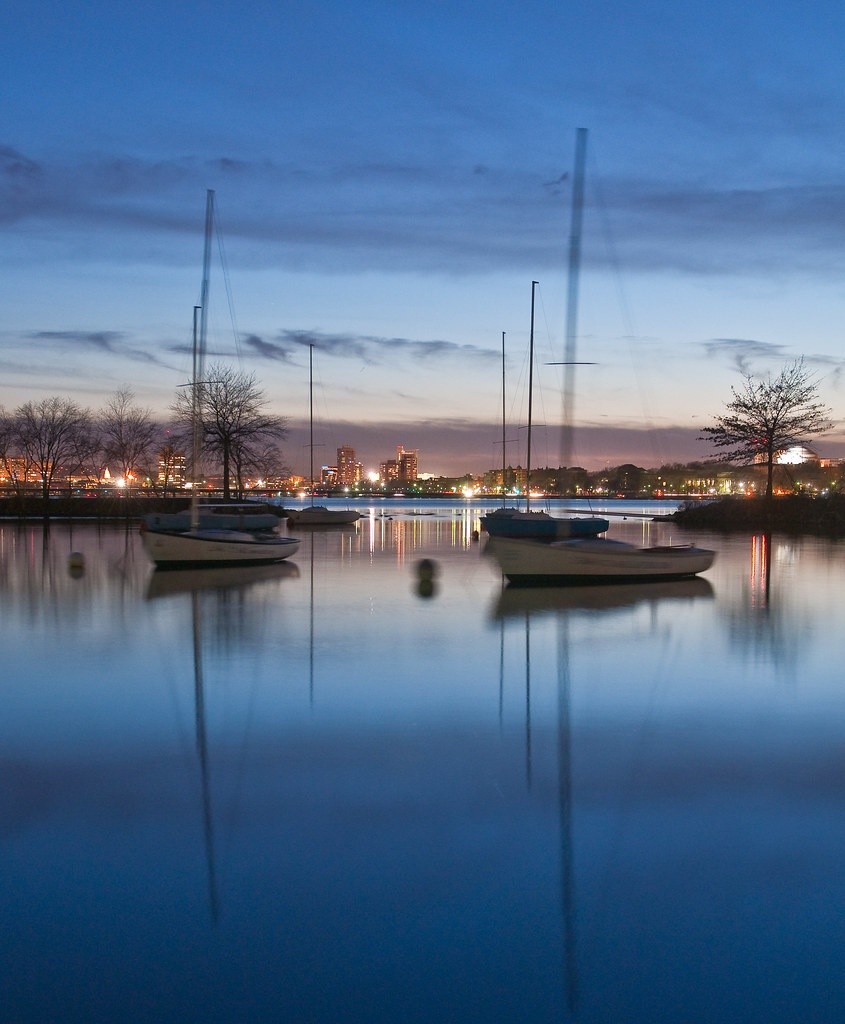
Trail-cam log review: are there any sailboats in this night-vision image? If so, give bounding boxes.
[280,343,361,525]
[479,279,610,538]
[140,187,302,566]
[484,120,717,587]
